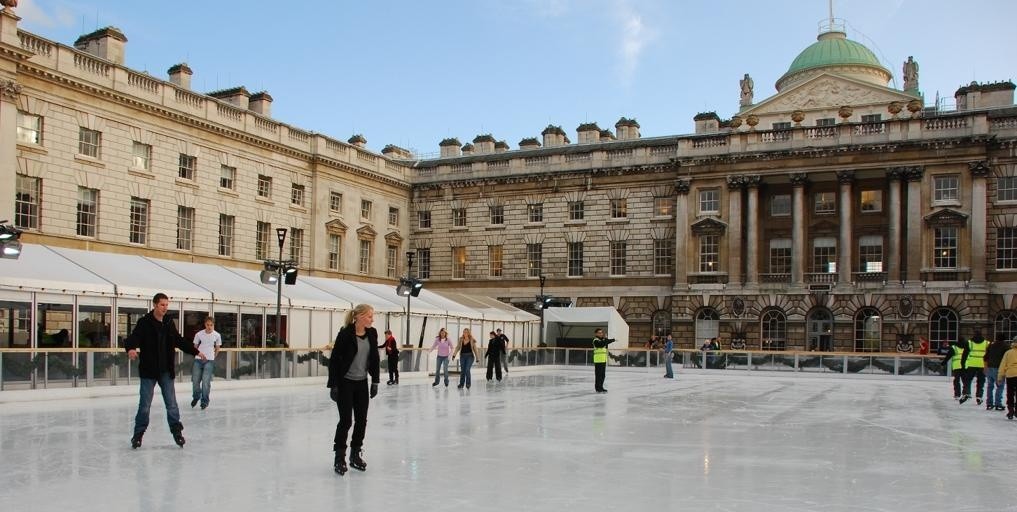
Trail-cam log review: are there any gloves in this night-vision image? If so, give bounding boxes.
[369,385,377,398]
[329,388,338,401]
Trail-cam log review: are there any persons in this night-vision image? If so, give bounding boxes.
[452,328,479,387]
[429,328,454,384]
[191,317,223,406]
[327,303,380,467]
[592,328,617,393]
[496,329,509,372]
[663,334,673,378]
[485,331,506,379]
[937,328,1017,417]
[919,337,928,354]
[377,330,399,382]
[124,293,205,447]
[701,338,720,350]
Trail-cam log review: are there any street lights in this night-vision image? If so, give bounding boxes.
[261,226,299,378]
[397,250,422,371]
[1,220,25,260]
[534,275,551,364]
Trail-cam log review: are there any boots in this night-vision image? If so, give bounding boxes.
[349,449,366,468]
[333,450,347,471]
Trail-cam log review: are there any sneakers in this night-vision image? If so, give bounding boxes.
[190,397,200,406]
[200,402,206,408]
[386,381,393,385]
[431,368,508,389]
[392,381,398,385]
[170,432,184,444]
[130,433,143,446]
[952,393,1017,420]
